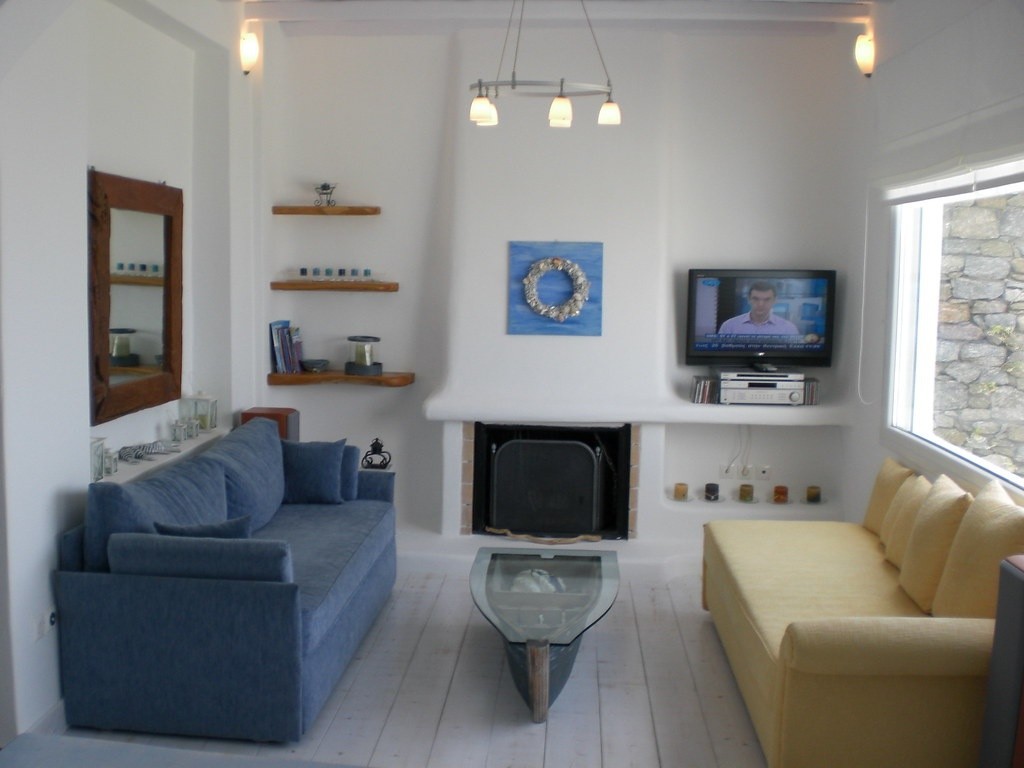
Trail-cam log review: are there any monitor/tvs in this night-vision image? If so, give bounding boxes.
[685,269,836,367]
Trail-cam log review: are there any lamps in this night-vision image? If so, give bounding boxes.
[467,1,621,129]
[240,32,258,75]
[855,35,876,77]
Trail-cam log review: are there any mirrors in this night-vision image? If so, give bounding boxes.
[90,169,185,423]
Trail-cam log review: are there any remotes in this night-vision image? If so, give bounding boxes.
[752,362,777,372]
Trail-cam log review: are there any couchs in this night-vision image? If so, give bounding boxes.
[49,417,397,744]
[702,460,1023,768]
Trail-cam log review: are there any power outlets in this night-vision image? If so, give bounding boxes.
[719,463,752,481]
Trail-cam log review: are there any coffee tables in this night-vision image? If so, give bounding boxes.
[469,547,622,724]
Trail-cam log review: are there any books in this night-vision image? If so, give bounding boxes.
[270,320,303,373]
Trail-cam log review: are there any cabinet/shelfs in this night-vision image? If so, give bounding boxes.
[268,204,415,388]
[107,272,171,377]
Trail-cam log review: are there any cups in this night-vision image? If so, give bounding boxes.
[674,482,688,499]
[774,485,788,502]
[740,484,753,501]
[805,486,821,501]
[705,483,719,500]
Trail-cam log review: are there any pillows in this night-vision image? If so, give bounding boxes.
[865,455,1023,620]
[154,514,252,539]
[280,437,349,505]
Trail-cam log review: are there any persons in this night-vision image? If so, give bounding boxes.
[719,281,798,334]
